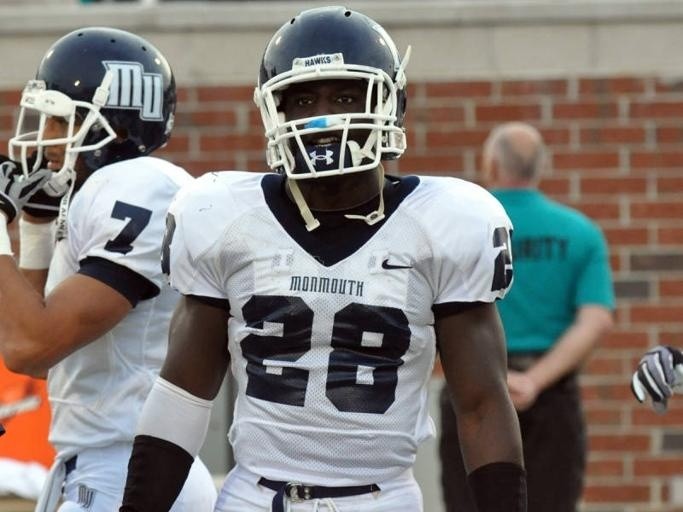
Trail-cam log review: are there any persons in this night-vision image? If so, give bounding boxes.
[438,120,619,511]
[0,25,218,511]
[119,5,533,512]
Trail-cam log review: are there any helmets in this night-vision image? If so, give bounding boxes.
[7,26,177,198]
[253,3,409,182]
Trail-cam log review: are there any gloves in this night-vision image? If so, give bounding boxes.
[19,152,72,218]
[0,154,53,223]
